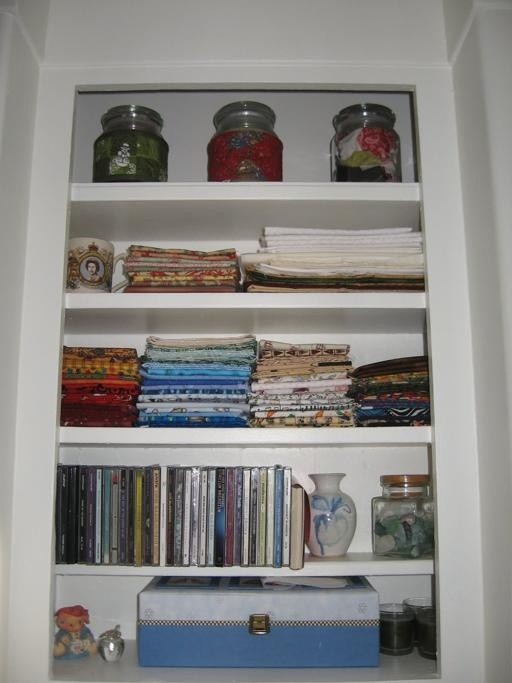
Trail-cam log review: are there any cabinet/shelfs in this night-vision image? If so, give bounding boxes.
[15,62,479,683]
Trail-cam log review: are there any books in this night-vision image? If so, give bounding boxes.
[53,464,309,570]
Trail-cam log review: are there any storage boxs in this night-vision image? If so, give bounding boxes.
[135,576,380,669]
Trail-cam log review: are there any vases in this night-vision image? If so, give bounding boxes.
[305,471,358,556]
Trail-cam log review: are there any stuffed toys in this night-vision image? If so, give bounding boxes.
[53,604,99,662]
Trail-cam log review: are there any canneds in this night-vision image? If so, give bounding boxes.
[92,105,168,182]
[206,100,282,182]
[330,104,403,180]
